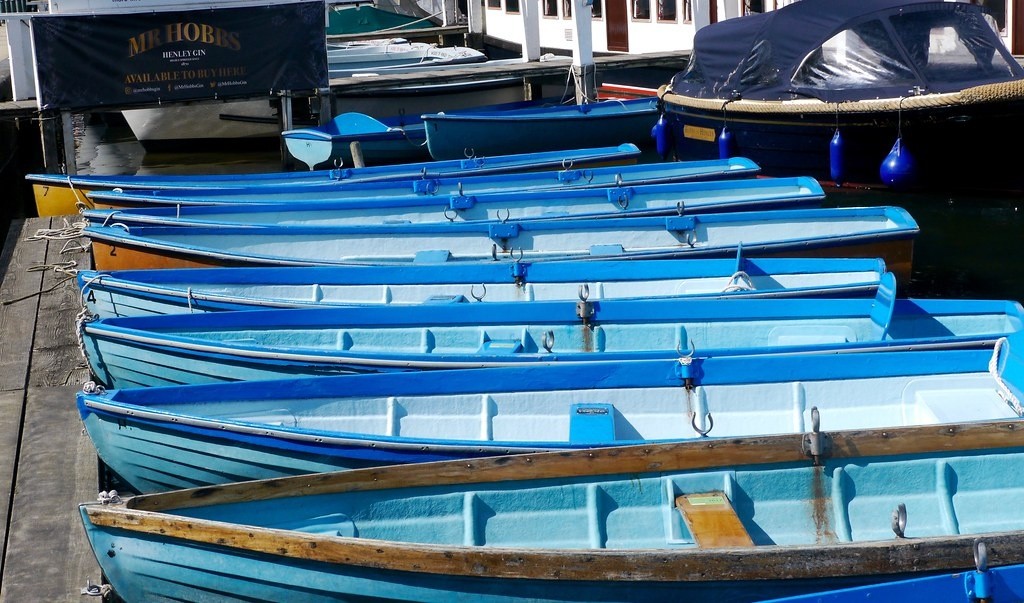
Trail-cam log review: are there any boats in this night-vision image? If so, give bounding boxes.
[26,143,922,283]
[594,1,1024,193]
[68,273,1024,390]
[66,413,1024,603]
[67,241,890,322]
[119,50,574,154]
[66,328,1024,494]
[325,0,488,79]
[418,93,665,161]
[281,92,573,171]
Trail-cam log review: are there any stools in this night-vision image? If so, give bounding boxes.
[589,244,622,254]
[422,296,463,304]
[569,403,615,442]
[474,338,520,353]
[412,250,449,265]
[674,491,755,550]
